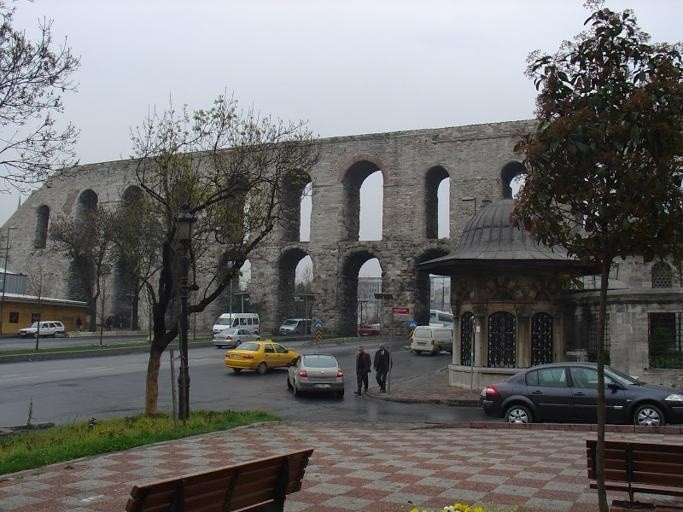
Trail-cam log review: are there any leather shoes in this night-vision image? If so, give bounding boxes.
[355,391,361,396]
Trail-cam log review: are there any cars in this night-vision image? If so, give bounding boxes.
[478,360,683,427]
[17,320,64,339]
[211,328,262,349]
[359,323,381,337]
[286,352,345,397]
[223,339,300,375]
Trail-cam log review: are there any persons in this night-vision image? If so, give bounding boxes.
[374,344,393,394]
[354,346,371,396]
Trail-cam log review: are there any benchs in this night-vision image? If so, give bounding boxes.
[123,446,315,512]
[585,437,683,510]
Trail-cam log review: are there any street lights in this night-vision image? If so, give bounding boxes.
[173,203,198,421]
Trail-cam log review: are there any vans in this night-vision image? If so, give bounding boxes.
[410,325,453,356]
[278,318,312,337]
[212,312,260,334]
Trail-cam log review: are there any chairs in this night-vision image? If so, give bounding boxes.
[572,367,589,388]
